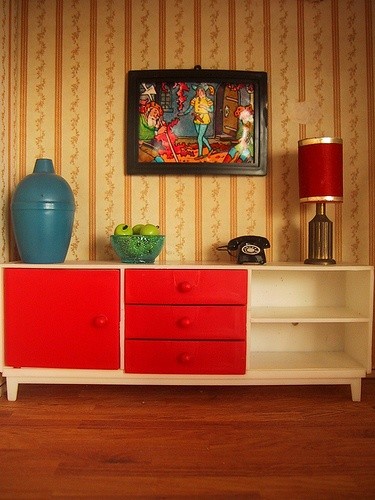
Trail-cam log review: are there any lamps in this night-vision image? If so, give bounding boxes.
[296,137,346,269]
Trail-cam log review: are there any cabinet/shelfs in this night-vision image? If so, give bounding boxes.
[0,264,373,402]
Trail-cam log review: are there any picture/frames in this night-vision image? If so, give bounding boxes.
[126,67,267,175]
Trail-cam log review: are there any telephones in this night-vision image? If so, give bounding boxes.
[217,236,270,265]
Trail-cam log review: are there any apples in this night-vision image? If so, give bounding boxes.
[132,224,144,235]
[139,224,159,235]
[114,224,133,236]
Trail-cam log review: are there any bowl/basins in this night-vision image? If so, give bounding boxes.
[109,235,167,264]
